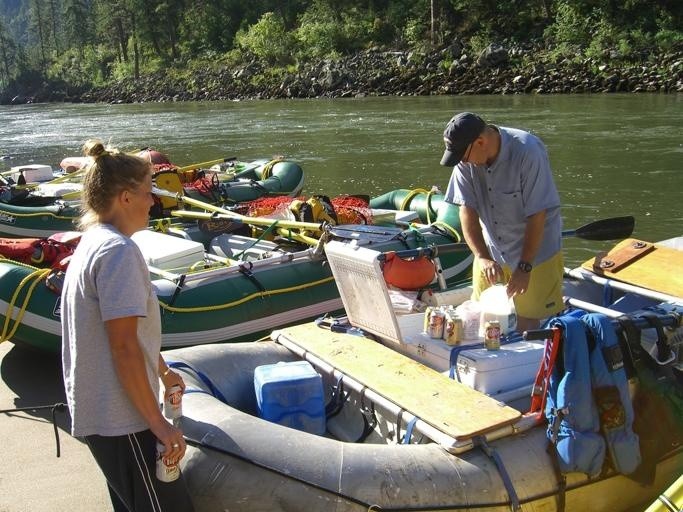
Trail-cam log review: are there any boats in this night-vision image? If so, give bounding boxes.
[153,236,683,512]
[0,186,488,353]
[1,156,309,237]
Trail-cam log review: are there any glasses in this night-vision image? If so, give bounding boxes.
[459,137,483,167]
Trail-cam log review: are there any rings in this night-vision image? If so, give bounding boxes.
[171,443,178,449]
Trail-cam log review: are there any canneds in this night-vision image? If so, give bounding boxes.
[156,441,180,482]
[165,386,182,419]
[485,320,500,351]
[424,305,462,346]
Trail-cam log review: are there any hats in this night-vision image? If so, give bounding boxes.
[439,112,485,168]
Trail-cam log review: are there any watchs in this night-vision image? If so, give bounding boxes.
[517,263,533,273]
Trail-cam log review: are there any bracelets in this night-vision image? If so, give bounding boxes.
[159,366,172,380]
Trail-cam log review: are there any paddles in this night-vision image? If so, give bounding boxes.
[160,209,403,243]
[378,216,635,261]
[18,145,238,207]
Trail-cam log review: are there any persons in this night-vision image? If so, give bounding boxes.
[55,139,187,512]
[437,110,567,332]
